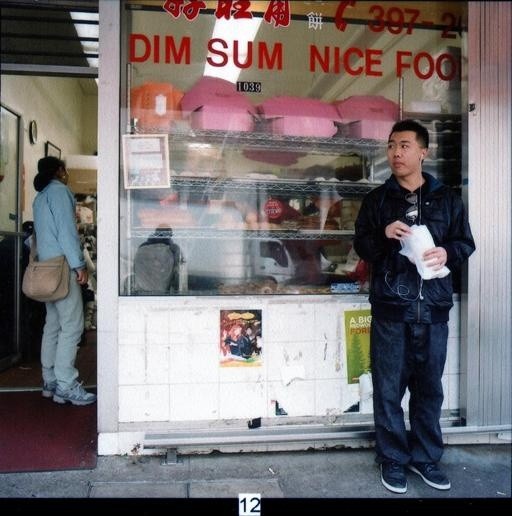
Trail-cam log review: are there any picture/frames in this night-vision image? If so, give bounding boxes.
[121,133,171,190]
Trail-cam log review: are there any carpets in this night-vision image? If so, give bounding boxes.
[1,389,99,475]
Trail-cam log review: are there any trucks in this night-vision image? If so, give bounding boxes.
[173,240,302,294]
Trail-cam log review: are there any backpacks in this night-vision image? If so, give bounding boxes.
[21,250,70,303]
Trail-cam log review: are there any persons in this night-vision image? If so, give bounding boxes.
[353,119,476,494]
[19,221,47,370]
[80,234,96,330]
[131,223,181,295]
[32,156,96,406]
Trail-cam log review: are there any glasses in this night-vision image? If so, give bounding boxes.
[405,192,419,223]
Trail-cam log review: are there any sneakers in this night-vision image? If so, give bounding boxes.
[41,380,97,406]
[406,459,452,490]
[380,460,408,494]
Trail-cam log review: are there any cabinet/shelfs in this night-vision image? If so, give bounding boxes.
[127,126,390,241]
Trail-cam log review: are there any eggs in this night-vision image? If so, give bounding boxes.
[217,212,281,230]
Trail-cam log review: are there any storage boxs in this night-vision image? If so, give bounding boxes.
[65,168,97,195]
